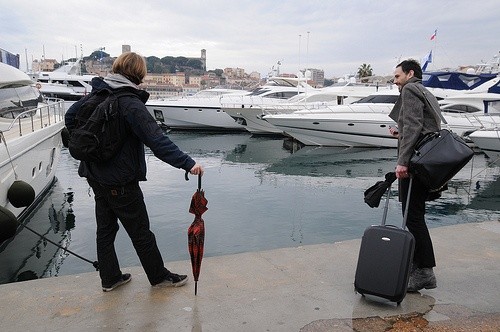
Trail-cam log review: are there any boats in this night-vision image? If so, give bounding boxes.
[143,75,500,151]
[0,61,67,248]
[34,59,99,97]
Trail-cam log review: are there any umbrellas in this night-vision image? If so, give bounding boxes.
[363,171,397,208]
[185,170,208,297]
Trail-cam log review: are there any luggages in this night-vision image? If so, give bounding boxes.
[355,171,416,306]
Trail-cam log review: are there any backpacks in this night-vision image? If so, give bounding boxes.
[61,88,139,162]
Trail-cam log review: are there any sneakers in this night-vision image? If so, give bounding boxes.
[153,272,188,288]
[103,273,132,291]
[407,268,436,291]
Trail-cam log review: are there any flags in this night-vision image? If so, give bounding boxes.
[429,30,436,42]
[422,51,432,72]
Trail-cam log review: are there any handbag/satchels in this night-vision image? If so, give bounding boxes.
[408,129,475,192]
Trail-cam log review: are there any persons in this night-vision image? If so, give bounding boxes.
[388,59,441,290]
[78,52,204,293]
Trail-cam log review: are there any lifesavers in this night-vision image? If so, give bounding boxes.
[36,83,41,89]
